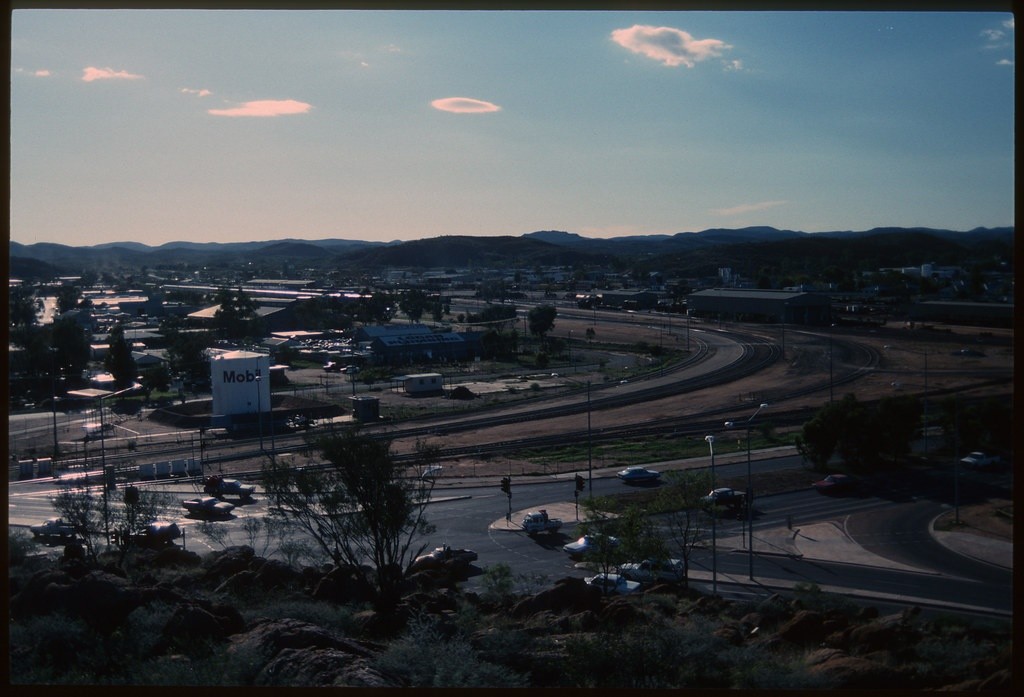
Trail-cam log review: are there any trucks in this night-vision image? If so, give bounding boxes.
[522,514,564,535]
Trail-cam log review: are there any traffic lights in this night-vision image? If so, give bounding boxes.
[576,474,585,491]
[501,476,511,493]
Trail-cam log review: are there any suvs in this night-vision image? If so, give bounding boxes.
[618,558,683,590]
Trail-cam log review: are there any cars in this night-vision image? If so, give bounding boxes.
[617,466,662,481]
[562,532,620,558]
[127,518,180,541]
[812,473,849,494]
[203,478,257,500]
[583,573,640,597]
[30,516,84,540]
[183,494,234,517]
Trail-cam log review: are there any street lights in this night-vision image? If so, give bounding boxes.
[890,380,962,528]
[828,321,837,407]
[724,404,772,581]
[705,434,716,594]
[51,396,64,461]
[448,284,788,364]
[881,344,930,459]
[99,382,143,548]
[550,372,593,493]
[256,375,264,451]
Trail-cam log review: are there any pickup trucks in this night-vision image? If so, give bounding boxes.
[701,488,747,511]
[960,450,1001,468]
